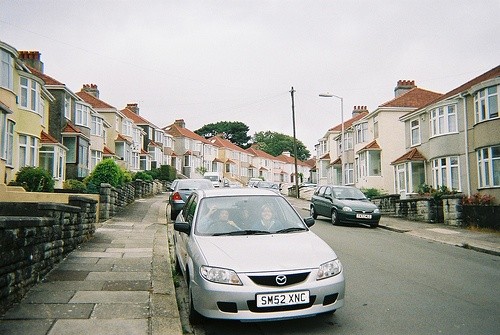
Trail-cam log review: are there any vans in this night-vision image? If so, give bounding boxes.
[204,171,222,188]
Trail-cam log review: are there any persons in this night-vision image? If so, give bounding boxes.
[250,204,285,232]
[198,204,243,233]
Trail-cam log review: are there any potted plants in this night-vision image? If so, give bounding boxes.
[416,183,436,198]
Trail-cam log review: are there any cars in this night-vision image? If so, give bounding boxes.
[167,179,180,204]
[310,185,381,229]
[225,176,282,197]
[168,179,215,220]
[171,188,346,328]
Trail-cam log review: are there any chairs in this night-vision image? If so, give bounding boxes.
[339,190,354,199]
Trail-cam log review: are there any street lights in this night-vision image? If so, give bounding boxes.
[318,92,344,186]
